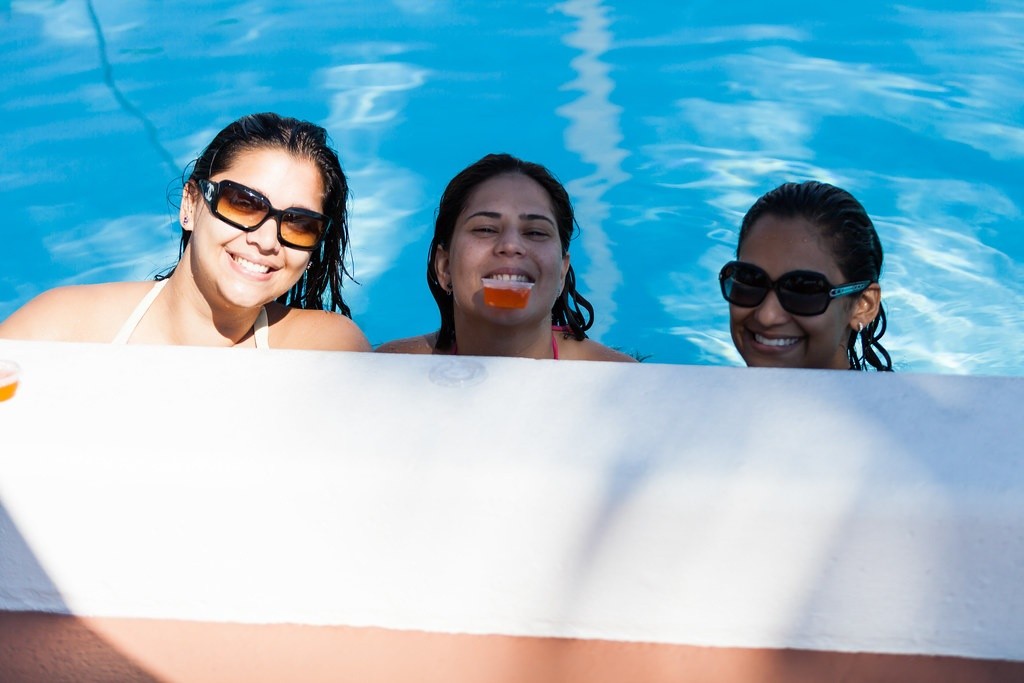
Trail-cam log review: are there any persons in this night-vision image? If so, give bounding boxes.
[719,181,894,372]
[0,112,373,353]
[374,153,642,363]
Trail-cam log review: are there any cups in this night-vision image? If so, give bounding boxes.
[481,277,536,308]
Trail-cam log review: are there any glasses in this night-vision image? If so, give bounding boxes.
[718,261,871,317]
[195,177,332,251]
